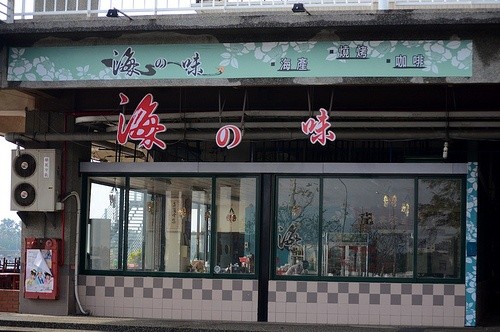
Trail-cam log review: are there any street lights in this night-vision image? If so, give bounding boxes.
[376,191,396,228]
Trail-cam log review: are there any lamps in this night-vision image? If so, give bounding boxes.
[106,8,133,20]
[292,3,311,15]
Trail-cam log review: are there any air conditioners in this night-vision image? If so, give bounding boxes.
[9,149,65,214]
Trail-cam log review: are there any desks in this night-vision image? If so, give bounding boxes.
[0,272,20,289]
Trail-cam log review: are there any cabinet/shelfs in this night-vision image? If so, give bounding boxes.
[87,218,111,270]
[325,231,369,276]
